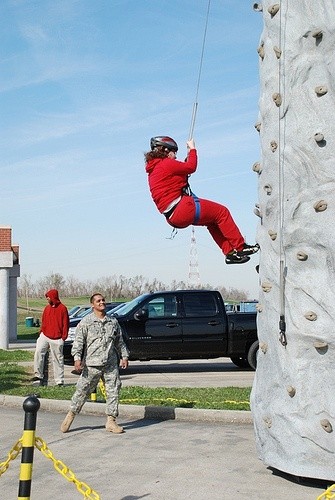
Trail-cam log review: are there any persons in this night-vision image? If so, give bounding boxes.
[61,294,129,433]
[30,289,69,386]
[145,136,260,265]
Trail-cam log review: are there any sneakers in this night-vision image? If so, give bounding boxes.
[238,243,260,255]
[225,251,250,265]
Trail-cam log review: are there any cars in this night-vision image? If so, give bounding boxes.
[68,301,165,323]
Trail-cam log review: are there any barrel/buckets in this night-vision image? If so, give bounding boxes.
[25,317,34,328]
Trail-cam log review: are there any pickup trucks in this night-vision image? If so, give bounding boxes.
[63,289,259,370]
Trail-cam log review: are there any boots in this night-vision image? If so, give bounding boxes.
[61,411,76,432]
[106,414,123,434]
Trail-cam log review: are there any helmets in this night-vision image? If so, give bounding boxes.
[150,136,178,152]
